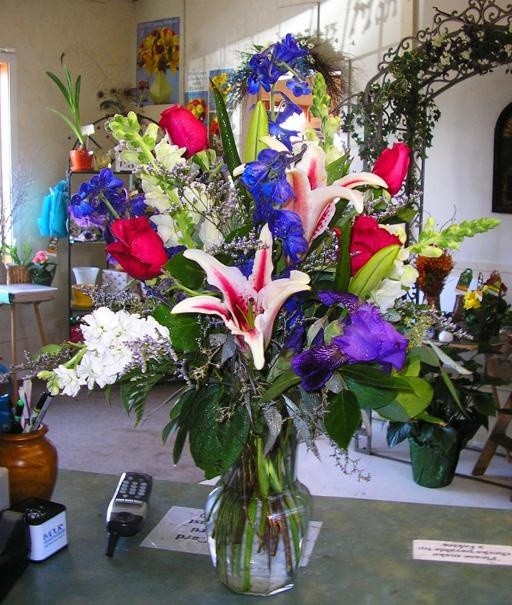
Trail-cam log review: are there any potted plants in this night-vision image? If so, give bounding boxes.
[4,247,28,283]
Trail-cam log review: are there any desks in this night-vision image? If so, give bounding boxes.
[0,285,65,419]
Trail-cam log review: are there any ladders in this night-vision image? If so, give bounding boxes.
[473,395,512,502]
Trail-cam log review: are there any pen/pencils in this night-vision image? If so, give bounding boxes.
[14,378,54,431]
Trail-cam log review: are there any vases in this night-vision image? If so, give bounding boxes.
[0,422,59,507]
[203,402,314,595]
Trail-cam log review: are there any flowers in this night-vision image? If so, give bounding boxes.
[39,32,505,402]
